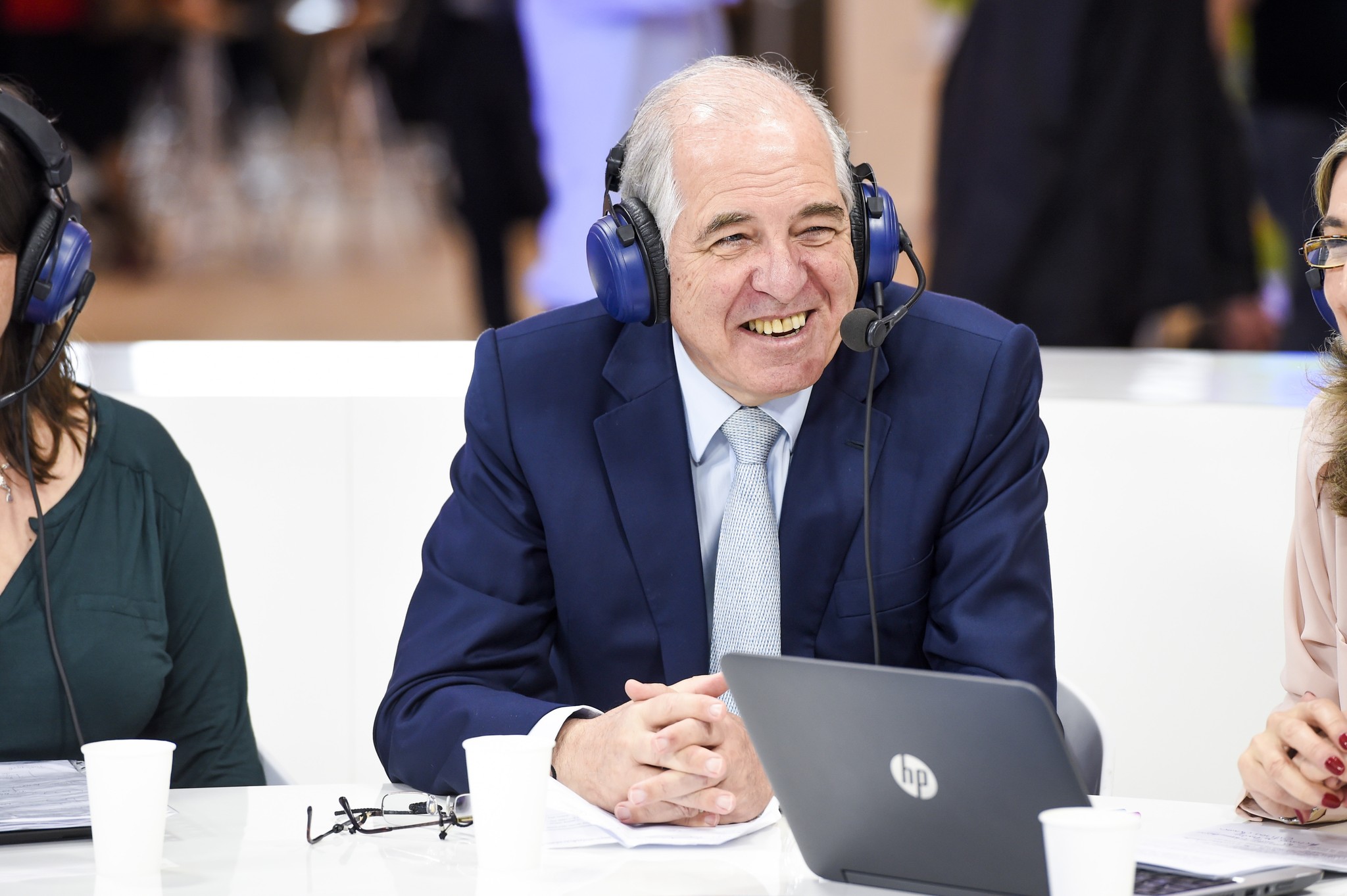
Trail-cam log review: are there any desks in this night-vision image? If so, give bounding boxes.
[0,775,1347,896]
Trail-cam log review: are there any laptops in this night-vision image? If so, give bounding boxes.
[720,651,1326,896]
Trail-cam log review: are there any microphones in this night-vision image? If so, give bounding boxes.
[840,224,925,353]
[0,270,96,410]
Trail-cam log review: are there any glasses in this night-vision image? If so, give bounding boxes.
[1298,235,1347,269]
[297,797,470,846]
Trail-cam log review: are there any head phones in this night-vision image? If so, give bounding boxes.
[1307,217,1340,335]
[586,127,898,328]
[0,87,90,350]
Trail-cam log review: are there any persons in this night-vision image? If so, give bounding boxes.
[1233,127,1345,832]
[1,82,267,839]
[8,3,1342,350]
[351,47,1067,832]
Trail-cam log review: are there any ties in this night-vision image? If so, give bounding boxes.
[709,410,787,717]
